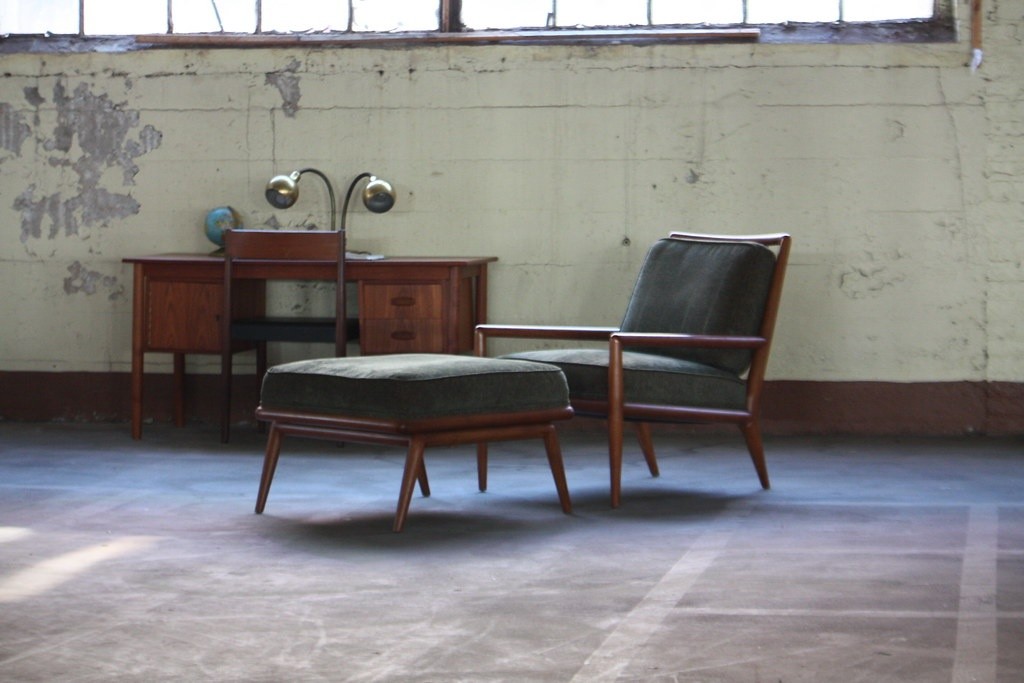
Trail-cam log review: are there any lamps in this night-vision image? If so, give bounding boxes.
[265,167,397,231]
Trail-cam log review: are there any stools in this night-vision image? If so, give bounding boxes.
[254,354,575,531]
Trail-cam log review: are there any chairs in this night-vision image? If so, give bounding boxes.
[473,232,791,506]
[221,228,361,443]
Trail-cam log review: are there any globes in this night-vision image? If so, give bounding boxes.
[205,205,244,257]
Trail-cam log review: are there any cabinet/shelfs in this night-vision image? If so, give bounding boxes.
[122,253,499,440]
